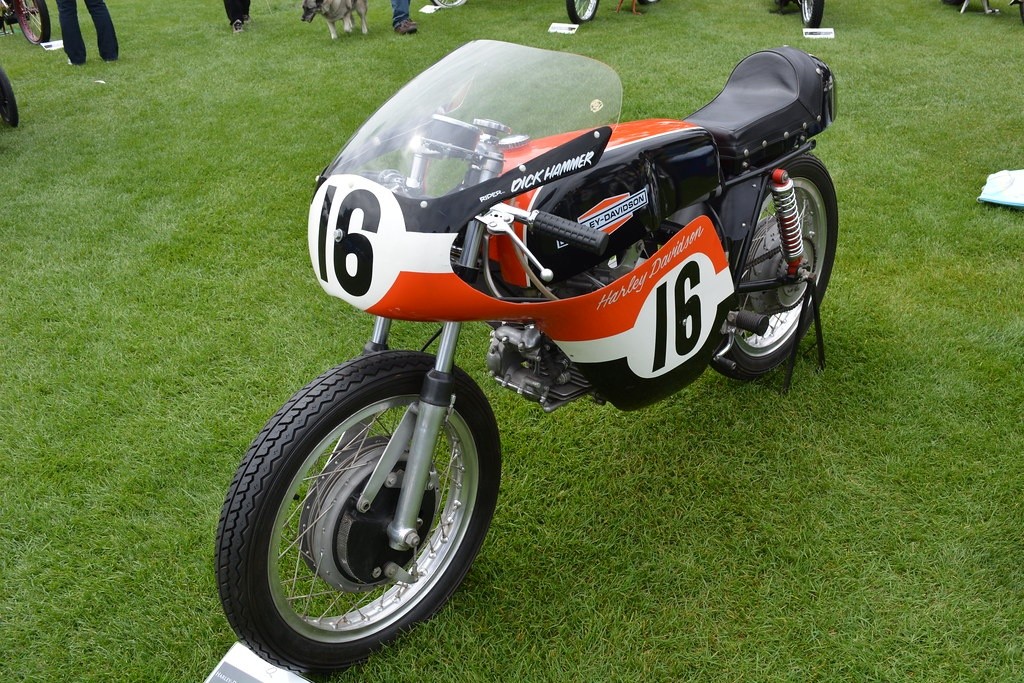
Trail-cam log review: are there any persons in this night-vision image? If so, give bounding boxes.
[390,0,417,34]
[223,0,251,34]
[56,0,118,65]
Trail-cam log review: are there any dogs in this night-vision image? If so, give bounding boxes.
[301,0,368,39]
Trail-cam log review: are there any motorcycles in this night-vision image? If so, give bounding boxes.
[431,0,466,8]
[774,0,824,28]
[0,1,51,44]
[0,70,19,126]
[566,0,658,24]
[942,0,1024,25]
[217,38,837,670]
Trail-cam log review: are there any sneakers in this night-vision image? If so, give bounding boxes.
[243,14,249,23]
[232,19,243,34]
[394,18,417,36]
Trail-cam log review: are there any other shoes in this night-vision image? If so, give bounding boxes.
[67,57,71,64]
